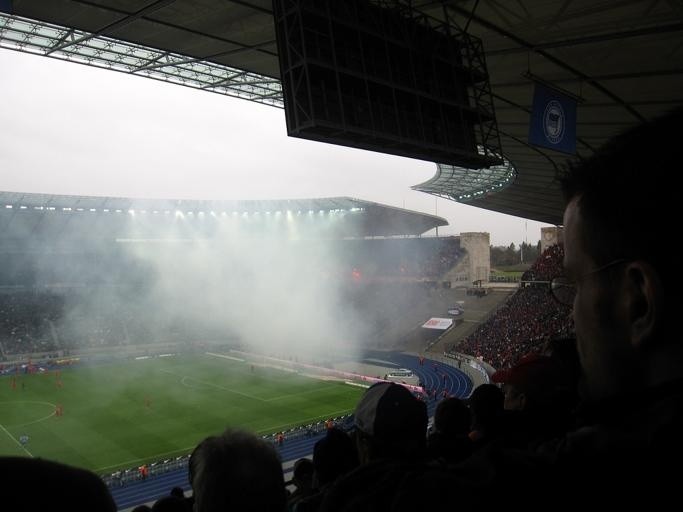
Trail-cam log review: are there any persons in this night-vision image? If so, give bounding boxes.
[0,455,117,510]
[149,334,327,374]
[110,427,275,512]
[0,293,66,391]
[55,401,63,416]
[18,433,29,448]
[276,374,412,512]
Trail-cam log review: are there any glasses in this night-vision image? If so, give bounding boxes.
[550,259,626,306]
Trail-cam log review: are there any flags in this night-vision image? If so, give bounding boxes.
[527,81,578,156]
